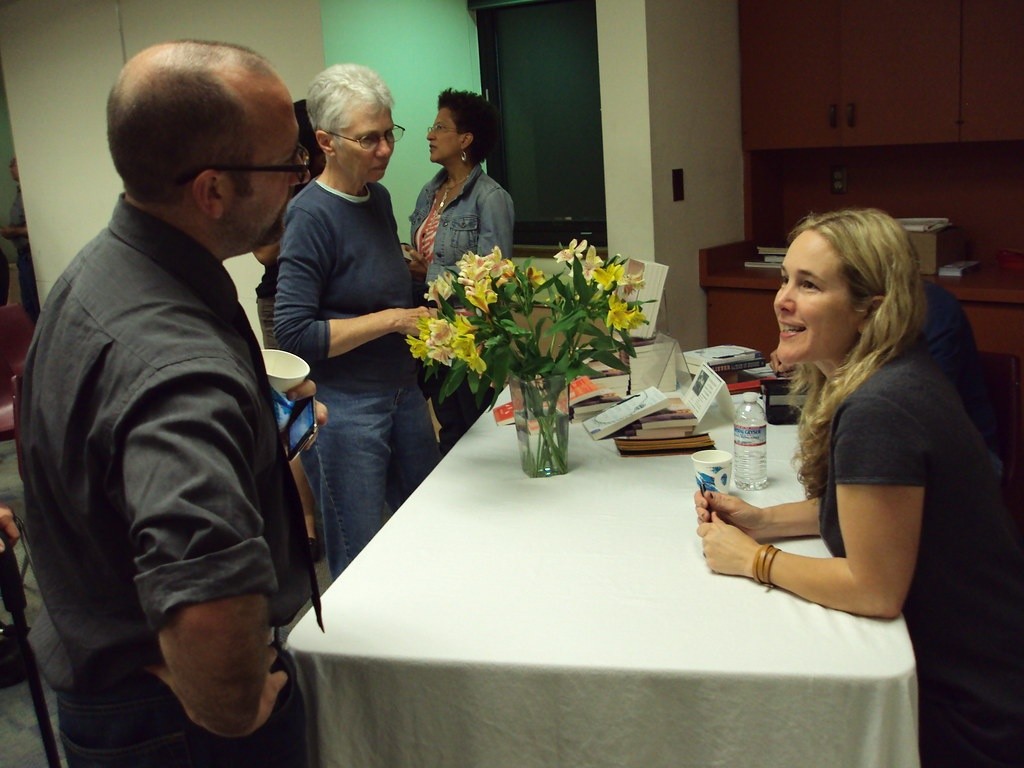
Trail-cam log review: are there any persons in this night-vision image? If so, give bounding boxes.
[694,208,1024,768]
[19,38,310,768]
[272,61,446,581]
[0,155,40,325]
[402,88,514,453]
[769,279,996,445]
[252,99,326,350]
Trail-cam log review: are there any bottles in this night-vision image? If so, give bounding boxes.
[733,391,769,492]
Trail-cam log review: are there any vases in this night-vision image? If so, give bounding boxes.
[509,374,570,478]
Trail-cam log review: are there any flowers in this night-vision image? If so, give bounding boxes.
[405,239,659,478]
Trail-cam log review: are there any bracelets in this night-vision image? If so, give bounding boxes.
[753,544,781,586]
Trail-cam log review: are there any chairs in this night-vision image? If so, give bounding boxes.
[0,303,36,480]
[955,351,1023,533]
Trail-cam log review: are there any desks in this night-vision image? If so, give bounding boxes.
[285,383,920,768]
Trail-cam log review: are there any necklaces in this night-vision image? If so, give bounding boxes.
[424,175,469,239]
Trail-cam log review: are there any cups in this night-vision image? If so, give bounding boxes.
[260,348,311,393]
[690,449,733,494]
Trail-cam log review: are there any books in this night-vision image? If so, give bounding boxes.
[625,258,669,338]
[582,386,715,453]
[493,376,622,426]
[745,247,789,269]
[678,345,765,375]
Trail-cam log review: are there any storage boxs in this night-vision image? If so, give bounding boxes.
[903,223,964,276]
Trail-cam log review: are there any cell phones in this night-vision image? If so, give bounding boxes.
[287,395,317,462]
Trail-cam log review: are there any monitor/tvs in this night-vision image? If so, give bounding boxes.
[478,0,608,249]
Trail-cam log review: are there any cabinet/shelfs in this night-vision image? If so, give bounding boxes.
[699,0,1023,353]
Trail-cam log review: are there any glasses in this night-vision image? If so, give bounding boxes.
[428,124,457,135]
[186,144,310,184]
[329,125,405,150]
